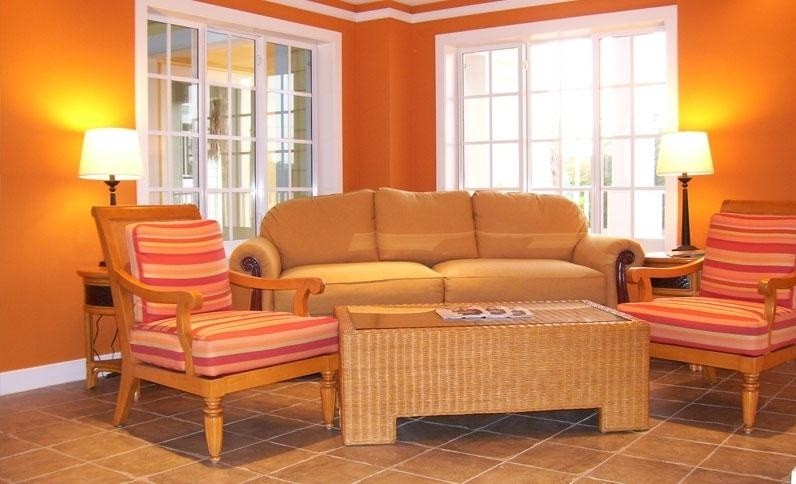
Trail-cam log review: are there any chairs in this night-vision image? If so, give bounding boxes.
[91,203,341,467]
[615,199,796,435]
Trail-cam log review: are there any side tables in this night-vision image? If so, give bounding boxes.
[76,263,142,403]
[643,248,706,372]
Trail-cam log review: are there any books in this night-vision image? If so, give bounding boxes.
[480,307,509,319]
[433,306,484,322]
[507,307,532,317]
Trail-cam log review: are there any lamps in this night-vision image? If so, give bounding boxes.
[76,127,148,269]
[653,129,716,252]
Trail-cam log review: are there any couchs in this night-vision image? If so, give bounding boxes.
[228,186,647,320]
